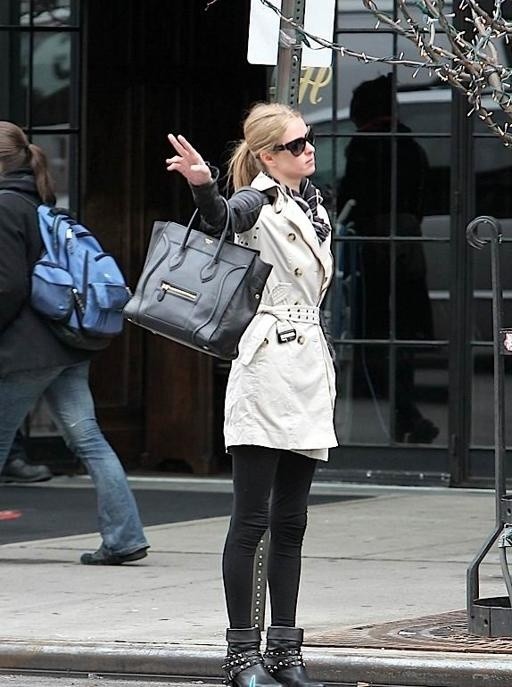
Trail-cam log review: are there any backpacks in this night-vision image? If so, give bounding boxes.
[0,188,133,352]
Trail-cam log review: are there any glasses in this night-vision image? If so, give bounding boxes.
[272,124,316,157]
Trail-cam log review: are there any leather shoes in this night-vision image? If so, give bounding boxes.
[0,457,53,484]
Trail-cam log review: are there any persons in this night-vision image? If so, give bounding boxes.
[1,119,151,566]
[336,71,440,444]
[2,424,54,486]
[165,100,343,686]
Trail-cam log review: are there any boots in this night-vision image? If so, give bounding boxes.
[262,625,325,687]
[221,627,284,687]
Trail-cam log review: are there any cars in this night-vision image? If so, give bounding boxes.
[299,87,511,365]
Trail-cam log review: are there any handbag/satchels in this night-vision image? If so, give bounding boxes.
[120,193,274,361]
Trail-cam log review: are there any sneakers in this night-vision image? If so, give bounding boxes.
[80,544,147,564]
